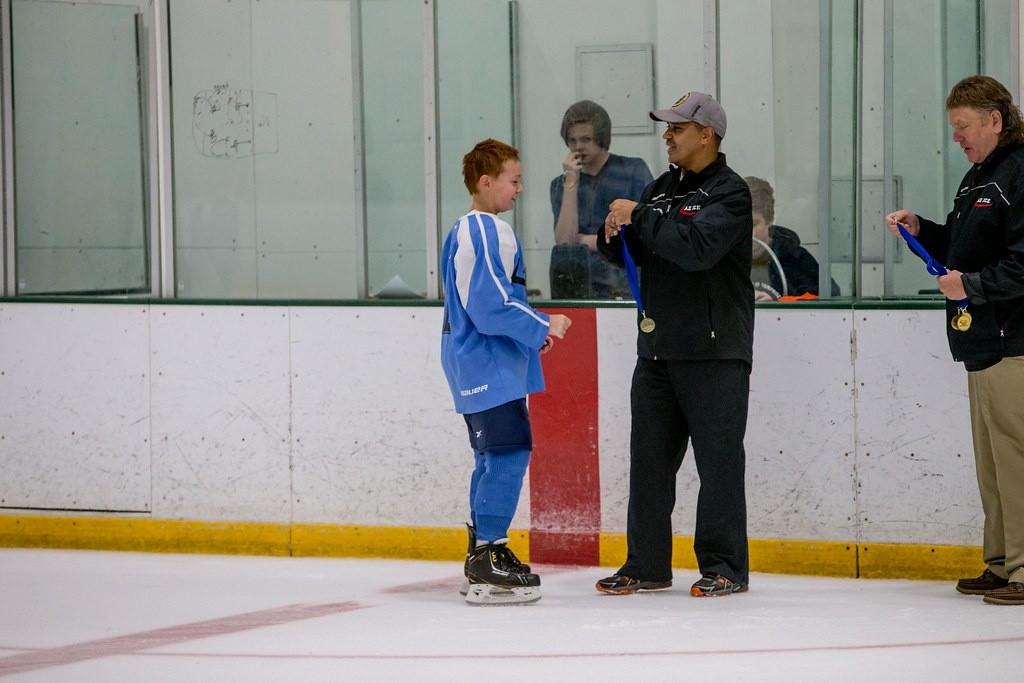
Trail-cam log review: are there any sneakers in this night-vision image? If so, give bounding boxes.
[465,524,541,605]
[691,571,750,598]
[460,524,531,595]
[956,569,1009,595]
[984,581,1024,605]
[595,571,674,595]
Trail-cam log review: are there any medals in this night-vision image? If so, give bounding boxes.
[640,318,656,333]
[951,311,972,331]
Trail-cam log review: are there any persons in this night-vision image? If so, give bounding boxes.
[441,137,572,608]
[887,74,1024,607]
[549,100,655,300]
[596,92,755,597]
[743,175,841,301]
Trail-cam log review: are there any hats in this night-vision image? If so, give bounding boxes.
[649,91,728,139]
